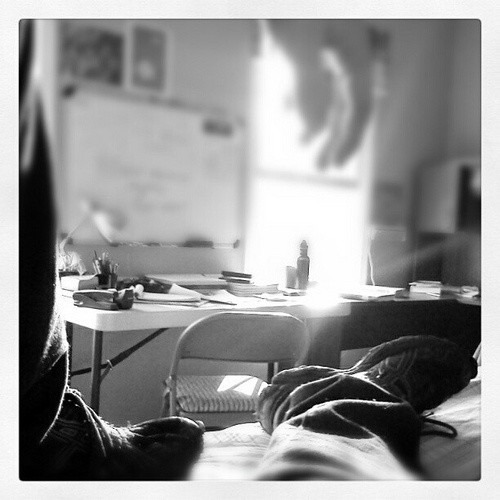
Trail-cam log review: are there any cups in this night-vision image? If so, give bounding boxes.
[285,265,297,289]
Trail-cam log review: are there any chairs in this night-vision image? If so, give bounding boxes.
[163,312,311,416]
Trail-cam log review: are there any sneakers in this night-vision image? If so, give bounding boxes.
[256,335,479,436]
[19,387,204,481]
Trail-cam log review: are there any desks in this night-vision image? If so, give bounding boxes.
[57,289,456,414]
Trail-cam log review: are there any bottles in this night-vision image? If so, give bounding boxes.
[298,240,310,290]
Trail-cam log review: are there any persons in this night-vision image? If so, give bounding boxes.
[19,19,478,481]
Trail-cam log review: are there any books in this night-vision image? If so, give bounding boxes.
[409,278,479,299]
[61,274,99,292]
[132,273,278,307]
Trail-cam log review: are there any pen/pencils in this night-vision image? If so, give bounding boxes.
[93,249,118,274]
